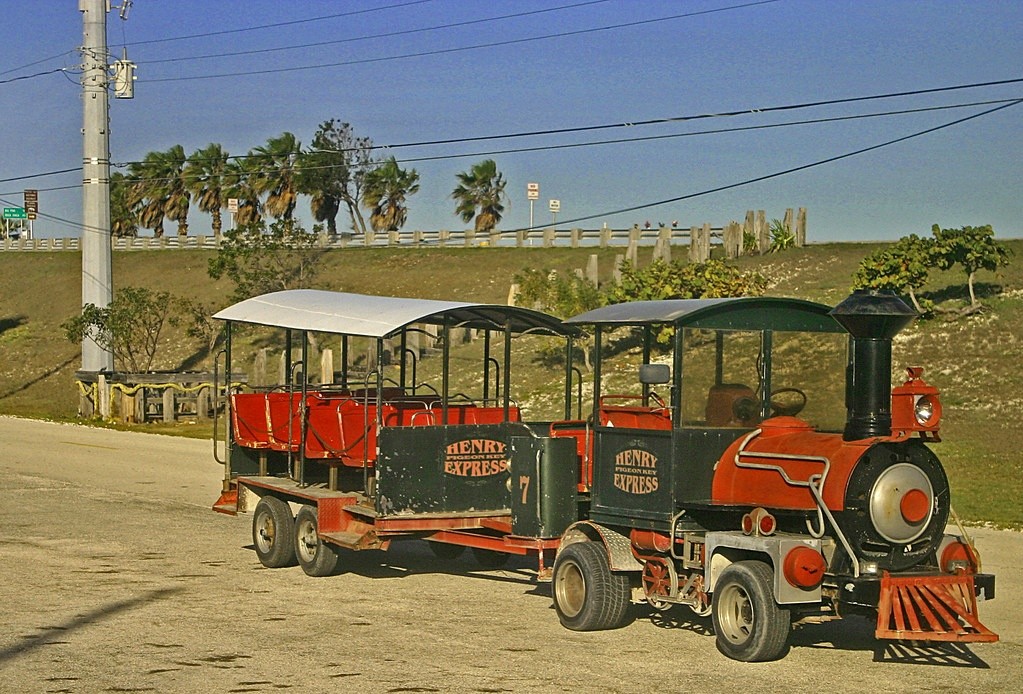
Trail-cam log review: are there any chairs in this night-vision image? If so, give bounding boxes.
[708,383,761,429]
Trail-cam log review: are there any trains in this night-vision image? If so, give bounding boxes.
[207,282,1002,663]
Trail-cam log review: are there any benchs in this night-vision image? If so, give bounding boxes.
[226,386,673,497]
